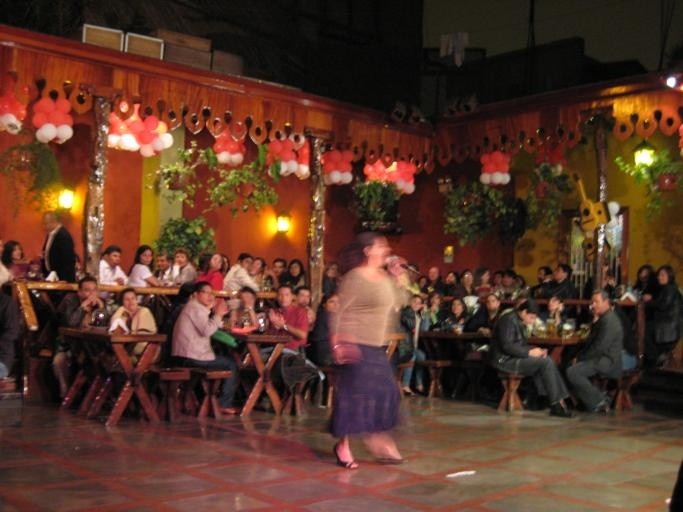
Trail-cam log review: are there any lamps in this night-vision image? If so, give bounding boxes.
[275,212,292,236]
[57,186,74,211]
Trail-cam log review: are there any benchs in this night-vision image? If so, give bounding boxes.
[180,368,232,422]
[139,368,191,425]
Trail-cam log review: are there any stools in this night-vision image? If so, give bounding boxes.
[347,334,639,411]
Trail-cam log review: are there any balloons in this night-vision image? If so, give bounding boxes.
[265,137,311,181]
[30,97,76,144]
[0,73,31,134]
[318,148,356,187]
[480,151,511,185]
[362,154,419,197]
[209,121,248,167]
[105,101,174,157]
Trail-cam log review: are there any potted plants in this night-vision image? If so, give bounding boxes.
[353,180,401,238]
[526,163,570,226]
[143,142,216,208]
[610,151,683,217]
[442,184,522,246]
[204,163,278,220]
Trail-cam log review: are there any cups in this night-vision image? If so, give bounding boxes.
[224,319,231,333]
[262,279,271,293]
[536,318,576,340]
[257,312,266,333]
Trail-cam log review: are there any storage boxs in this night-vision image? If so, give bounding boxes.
[124,32,164,64]
[164,45,213,68]
[73,24,124,51]
[157,28,213,53]
[214,49,245,77]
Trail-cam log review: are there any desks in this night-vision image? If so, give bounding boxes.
[57,327,162,429]
[225,333,290,418]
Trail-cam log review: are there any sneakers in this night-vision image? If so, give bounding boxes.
[219,407,241,415]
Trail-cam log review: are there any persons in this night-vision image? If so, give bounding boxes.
[107,287,159,368]
[328,229,412,470]
[0,208,84,396]
[395,262,682,398]
[88,242,223,305]
[563,288,623,413]
[172,281,237,414]
[156,281,197,369]
[51,275,110,404]
[222,252,342,410]
[490,300,572,417]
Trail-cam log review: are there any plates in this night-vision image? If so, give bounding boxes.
[158,281,175,286]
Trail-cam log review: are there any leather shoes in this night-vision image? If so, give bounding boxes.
[550,407,576,418]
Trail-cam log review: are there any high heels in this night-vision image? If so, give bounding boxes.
[333,442,359,470]
